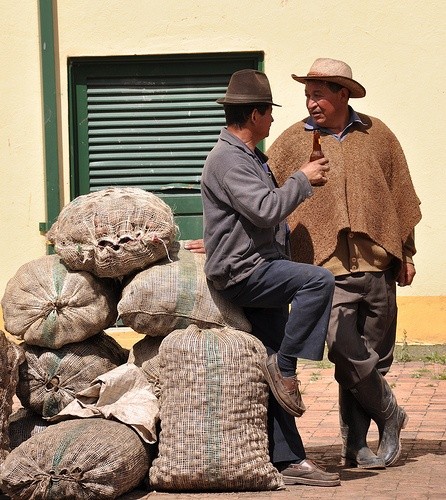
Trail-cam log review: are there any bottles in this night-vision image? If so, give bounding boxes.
[310,130,325,186]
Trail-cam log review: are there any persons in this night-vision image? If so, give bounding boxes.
[185,58,422,468]
[201,69,341,487]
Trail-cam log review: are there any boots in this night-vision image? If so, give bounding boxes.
[338,369,409,469]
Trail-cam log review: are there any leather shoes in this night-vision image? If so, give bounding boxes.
[279,457,341,487]
[261,353,307,418]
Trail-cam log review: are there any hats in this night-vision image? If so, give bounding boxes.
[291,57,367,98]
[217,69,282,107]
